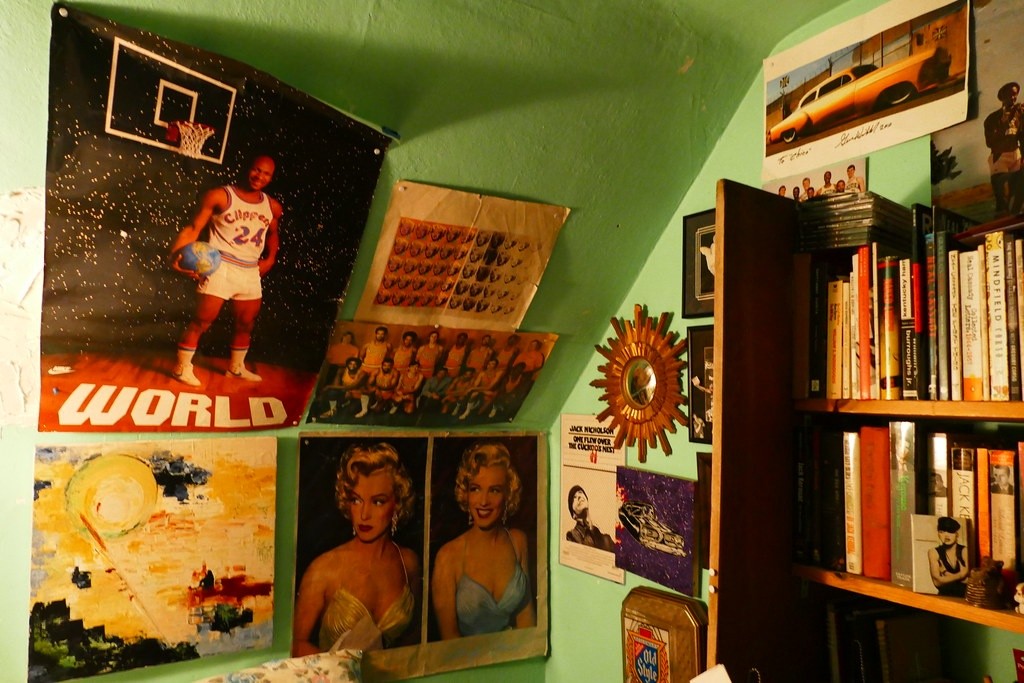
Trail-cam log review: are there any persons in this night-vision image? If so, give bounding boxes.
[928,516,968,596]
[431,442,537,639]
[292,441,422,657]
[565,484,616,553]
[318,326,544,419]
[953,449,972,471]
[167,154,282,385]
[983,82,1024,218]
[991,465,1014,495]
[376,219,542,315]
[928,472,947,497]
[778,165,865,203]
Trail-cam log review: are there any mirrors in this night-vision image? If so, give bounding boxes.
[589,304,687,463]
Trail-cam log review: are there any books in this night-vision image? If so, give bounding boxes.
[826,605,942,683]
[796,421,1024,595]
[810,204,1024,402]
[817,191,909,250]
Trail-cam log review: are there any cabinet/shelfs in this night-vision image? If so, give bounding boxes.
[705,179,1024,683]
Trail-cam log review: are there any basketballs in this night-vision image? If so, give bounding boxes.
[179,241,221,276]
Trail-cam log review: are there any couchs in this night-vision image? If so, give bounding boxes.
[195,648,363,683]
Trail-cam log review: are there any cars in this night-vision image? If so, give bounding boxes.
[768,42,950,146]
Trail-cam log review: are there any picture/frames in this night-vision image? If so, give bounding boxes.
[681,207,716,319]
[686,324,714,445]
